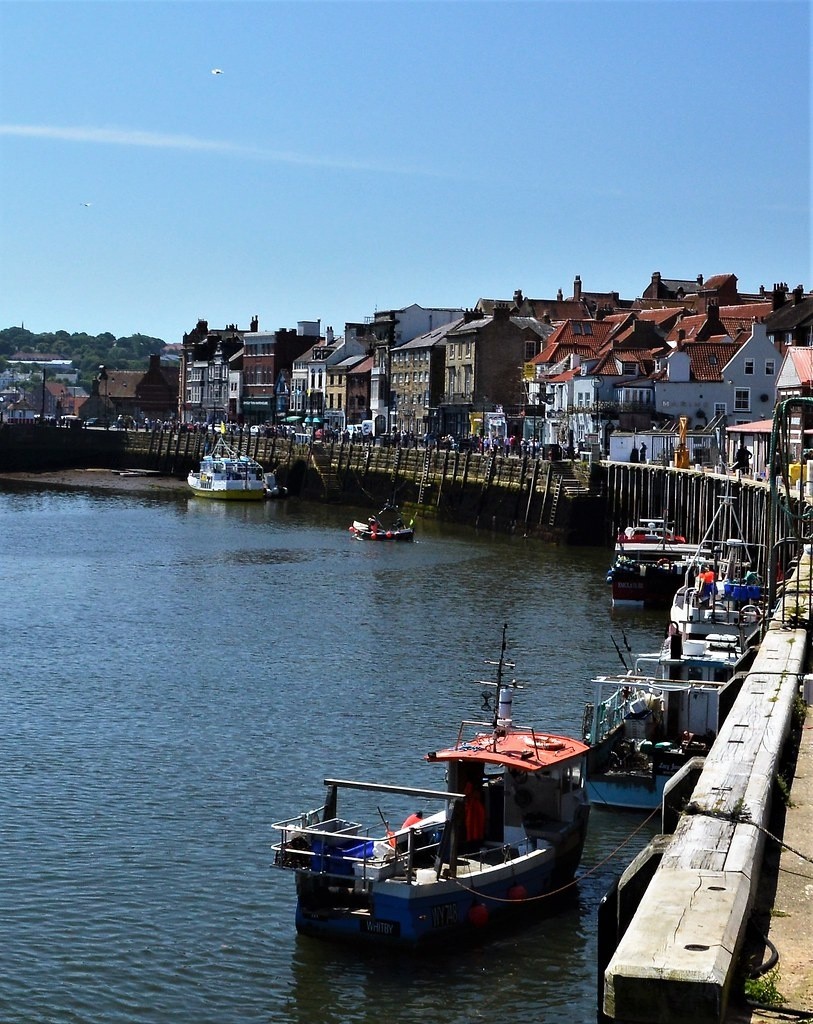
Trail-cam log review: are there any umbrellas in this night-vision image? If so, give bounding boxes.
[280,416,302,422]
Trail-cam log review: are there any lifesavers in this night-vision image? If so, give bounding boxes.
[524,735,562,749]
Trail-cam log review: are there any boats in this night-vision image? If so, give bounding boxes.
[270,622,593,947]
[348,500,414,540]
[577,477,776,811]
[187,434,267,500]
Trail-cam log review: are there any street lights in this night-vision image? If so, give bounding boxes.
[591,376,604,433]
[528,391,540,458]
[248,394,255,436]
[481,394,490,456]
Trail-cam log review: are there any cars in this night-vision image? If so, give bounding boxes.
[112,422,120,428]
[83,418,99,427]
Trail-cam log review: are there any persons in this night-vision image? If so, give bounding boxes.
[639,441,648,464]
[732,445,813,477]
[402,811,422,829]
[114,415,569,459]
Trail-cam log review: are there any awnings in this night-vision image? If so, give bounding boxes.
[304,417,324,423]
